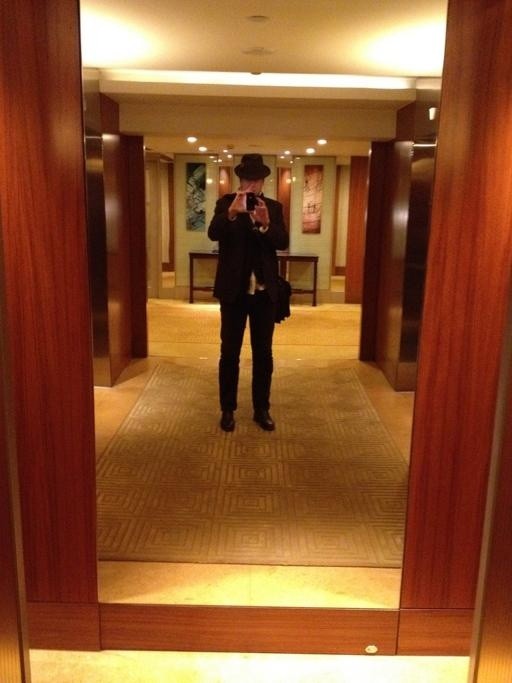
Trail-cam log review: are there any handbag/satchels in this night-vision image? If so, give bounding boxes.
[252,274,291,324]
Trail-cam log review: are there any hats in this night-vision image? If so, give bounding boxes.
[234,154,271,180]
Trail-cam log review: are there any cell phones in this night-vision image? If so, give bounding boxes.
[246,192,255,210]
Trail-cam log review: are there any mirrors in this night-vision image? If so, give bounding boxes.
[68,0,451,618]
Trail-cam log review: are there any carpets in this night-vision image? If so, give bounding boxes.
[89,351,414,570]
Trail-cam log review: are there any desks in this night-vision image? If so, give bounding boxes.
[184,244,322,309]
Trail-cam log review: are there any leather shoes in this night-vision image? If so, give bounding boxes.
[252,407,276,431]
[220,409,235,432]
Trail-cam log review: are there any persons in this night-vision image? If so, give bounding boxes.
[205,149,290,434]
